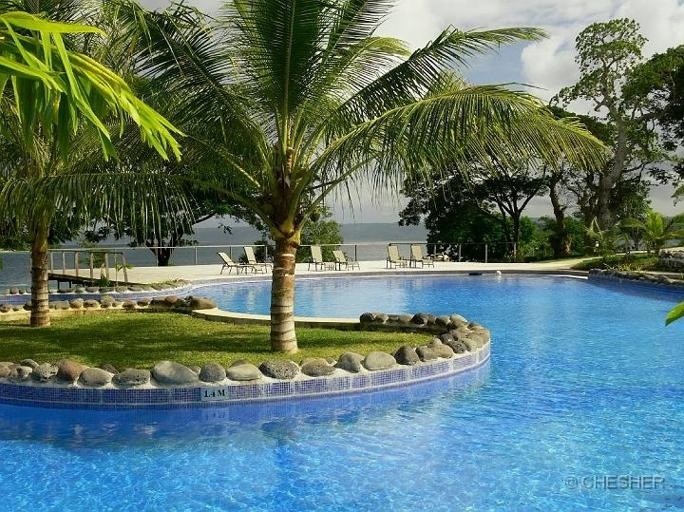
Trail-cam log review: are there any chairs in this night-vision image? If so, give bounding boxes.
[385,245,407,269]
[332,250,360,271]
[216,252,258,275]
[308,245,334,271]
[408,244,435,268]
[243,246,273,274]
